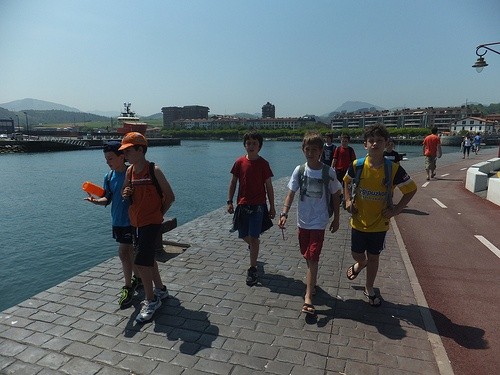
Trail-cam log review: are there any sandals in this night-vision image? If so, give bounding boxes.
[304,283,316,295]
[302,303,315,315]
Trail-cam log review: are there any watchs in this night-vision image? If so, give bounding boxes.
[280,213,288,219]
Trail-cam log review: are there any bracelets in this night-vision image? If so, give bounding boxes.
[227,201,233,204]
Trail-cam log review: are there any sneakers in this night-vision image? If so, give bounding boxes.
[246,266,258,284]
[136,294,162,322]
[130,275,141,289]
[114,286,135,306]
[153,285,169,299]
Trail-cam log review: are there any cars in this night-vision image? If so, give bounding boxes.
[78,134,122,139]
[0,133,23,140]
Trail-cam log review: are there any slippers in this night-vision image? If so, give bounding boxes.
[362,286,381,307]
[346,259,368,281]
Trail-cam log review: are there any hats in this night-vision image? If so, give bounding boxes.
[118,132,148,151]
[104,142,121,151]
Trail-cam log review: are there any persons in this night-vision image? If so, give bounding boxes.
[227,131,276,286]
[84,141,145,310]
[278,131,343,315]
[320,133,337,167]
[331,133,356,211]
[461,132,472,159]
[423,127,442,181]
[473,132,481,156]
[117,132,175,323]
[343,123,417,308]
[384,138,403,197]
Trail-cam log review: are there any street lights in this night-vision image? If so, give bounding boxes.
[16,115,20,133]
[23,112,28,135]
